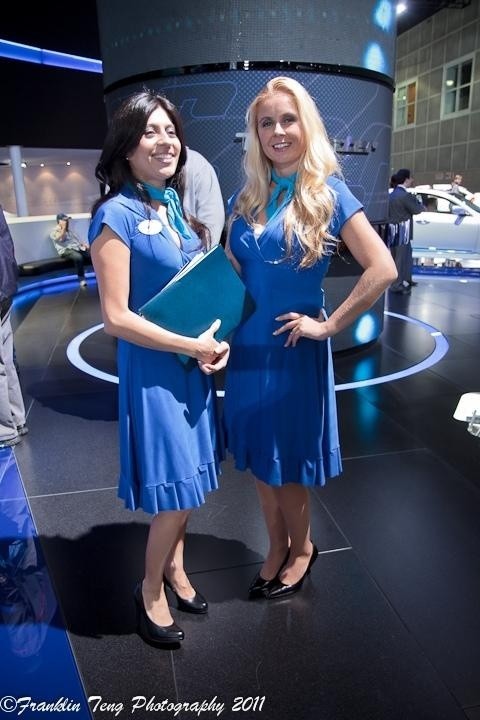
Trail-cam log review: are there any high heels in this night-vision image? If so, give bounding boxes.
[248,540,318,598]
[134,574,207,644]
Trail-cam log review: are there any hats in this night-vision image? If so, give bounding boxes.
[58,213,71,222]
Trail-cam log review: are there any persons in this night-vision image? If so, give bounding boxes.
[50,212,91,287]
[180,146,225,254]
[87,90,232,644]
[0,203,28,448]
[0,448,61,693]
[223,77,398,599]
[387,169,462,292]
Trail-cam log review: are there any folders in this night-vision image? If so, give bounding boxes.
[137,244,257,363]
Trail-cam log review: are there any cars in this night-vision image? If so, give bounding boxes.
[388,183,479,256]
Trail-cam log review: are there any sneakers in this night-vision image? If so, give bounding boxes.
[80,280,87,286]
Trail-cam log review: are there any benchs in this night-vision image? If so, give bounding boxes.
[19,248,93,276]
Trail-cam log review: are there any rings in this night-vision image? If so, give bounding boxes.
[289,332,295,337]
